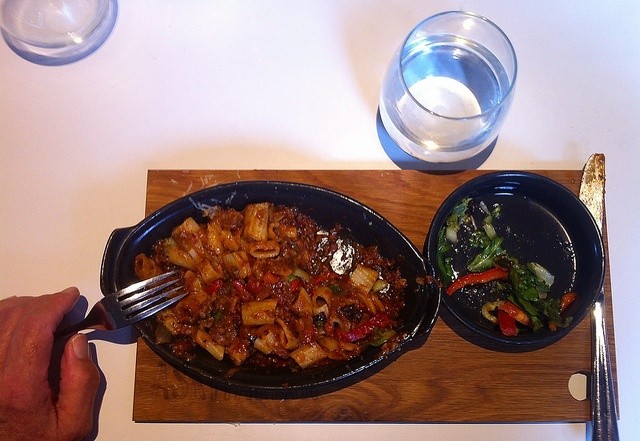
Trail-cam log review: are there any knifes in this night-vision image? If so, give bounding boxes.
[579,154,620,441]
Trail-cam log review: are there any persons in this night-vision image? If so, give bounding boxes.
[0,286,101,441]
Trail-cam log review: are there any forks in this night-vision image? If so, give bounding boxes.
[57,271,189,337]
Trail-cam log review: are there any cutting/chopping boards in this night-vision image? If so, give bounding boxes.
[132,170,619,423]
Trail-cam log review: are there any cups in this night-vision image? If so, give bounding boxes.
[378,10,517,164]
[0,1,118,65]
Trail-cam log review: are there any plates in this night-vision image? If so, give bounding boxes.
[99,181,442,399]
[423,171,606,353]
